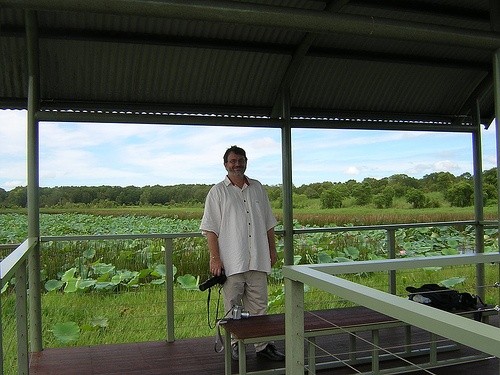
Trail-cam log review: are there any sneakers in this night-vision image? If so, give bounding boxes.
[256,344,285,362]
[232,343,248,360]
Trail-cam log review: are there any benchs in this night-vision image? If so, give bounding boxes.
[216,304,500,375]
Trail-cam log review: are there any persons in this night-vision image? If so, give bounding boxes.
[199,146,284,360]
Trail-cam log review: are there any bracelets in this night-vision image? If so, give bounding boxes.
[209,256,220,259]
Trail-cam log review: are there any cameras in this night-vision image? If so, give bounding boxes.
[199,268,227,292]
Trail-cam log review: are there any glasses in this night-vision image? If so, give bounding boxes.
[227,159,246,164]
